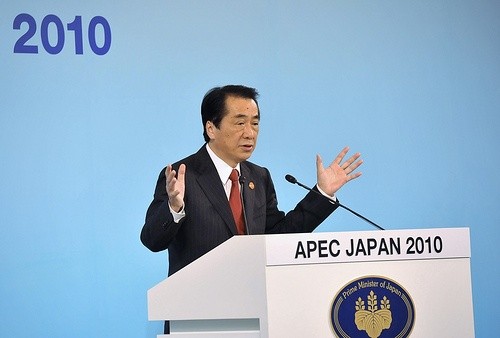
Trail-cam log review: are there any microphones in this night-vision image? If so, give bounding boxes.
[285,175,385,230]
[239,176,250,235]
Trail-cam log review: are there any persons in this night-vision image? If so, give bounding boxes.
[140,85,363,334]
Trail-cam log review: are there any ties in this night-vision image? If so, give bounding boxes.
[228,169,244,235]
